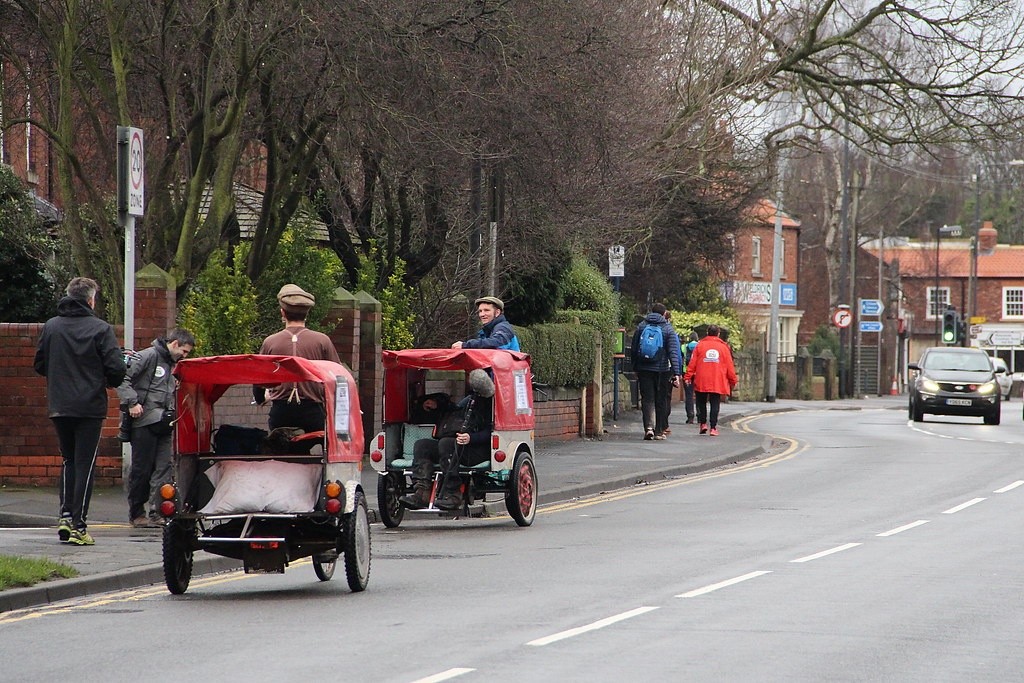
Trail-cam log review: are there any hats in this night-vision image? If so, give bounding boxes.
[277,284,315,314]
[475,297,503,312]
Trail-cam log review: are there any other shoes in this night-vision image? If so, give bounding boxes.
[663,428,671,434]
[686,419,693,424]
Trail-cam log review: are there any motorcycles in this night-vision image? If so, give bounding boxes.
[159,352,372,594]
[369,348,539,527]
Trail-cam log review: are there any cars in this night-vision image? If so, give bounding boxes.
[989,356,1015,401]
[22,190,70,241]
[907,346,1007,426]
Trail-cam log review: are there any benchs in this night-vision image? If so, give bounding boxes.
[390,422,492,473]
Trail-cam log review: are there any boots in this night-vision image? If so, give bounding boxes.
[399,479,432,508]
[434,485,461,510]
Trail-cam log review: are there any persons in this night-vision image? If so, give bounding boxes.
[683,324,737,436]
[622,316,644,412]
[32,277,126,546]
[253,284,344,435]
[452,297,520,381]
[680,332,702,425]
[631,302,680,440]
[114,329,197,528]
[399,374,493,512]
[661,310,684,435]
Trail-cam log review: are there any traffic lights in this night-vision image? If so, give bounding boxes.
[942,310,956,343]
[957,318,967,342]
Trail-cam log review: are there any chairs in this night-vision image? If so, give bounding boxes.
[930,356,947,369]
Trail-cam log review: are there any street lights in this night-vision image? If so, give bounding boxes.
[973,160,1024,317]
[935,224,963,347]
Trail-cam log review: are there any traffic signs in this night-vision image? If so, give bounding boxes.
[861,299,885,317]
[859,321,884,332]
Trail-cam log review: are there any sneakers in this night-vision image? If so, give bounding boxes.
[130,514,149,528]
[710,429,718,435]
[69,527,95,545]
[654,434,666,439]
[700,424,707,434]
[58,517,73,540]
[644,428,653,440]
[147,513,169,528]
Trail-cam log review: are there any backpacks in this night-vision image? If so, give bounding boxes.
[637,323,669,363]
[686,342,697,366]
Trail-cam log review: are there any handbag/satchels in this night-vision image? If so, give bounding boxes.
[209,424,268,455]
[410,392,451,424]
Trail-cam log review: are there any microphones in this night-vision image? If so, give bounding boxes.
[468,368,495,398]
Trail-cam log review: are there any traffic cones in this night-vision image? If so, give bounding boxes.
[889,376,900,396]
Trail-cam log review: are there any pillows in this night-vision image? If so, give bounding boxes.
[194,459,324,515]
